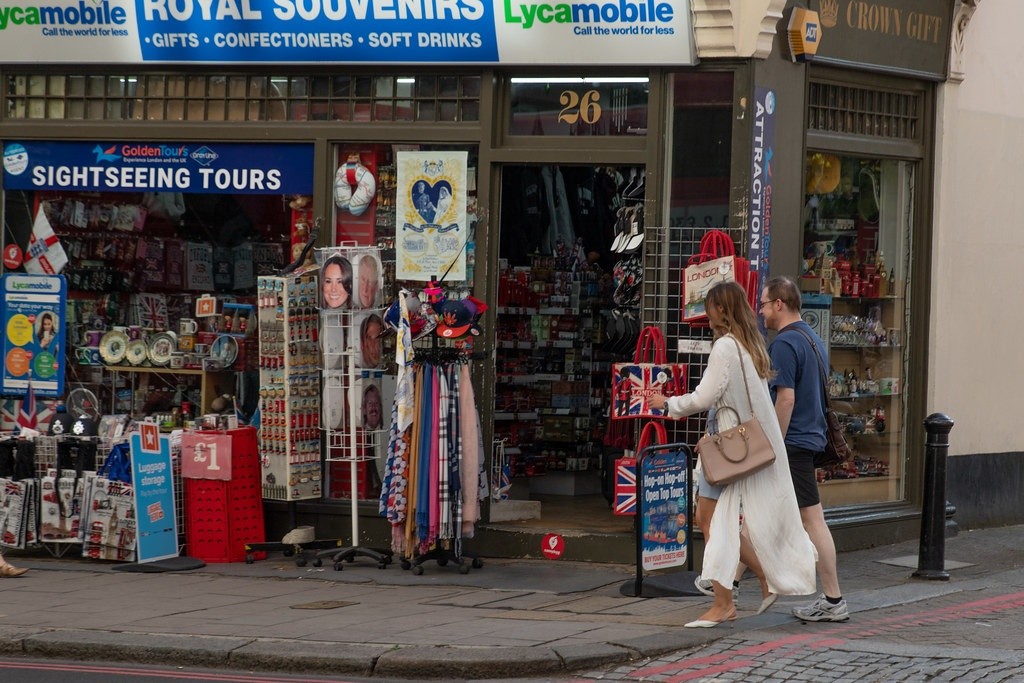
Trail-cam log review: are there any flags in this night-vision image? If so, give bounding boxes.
[22,202,69,276]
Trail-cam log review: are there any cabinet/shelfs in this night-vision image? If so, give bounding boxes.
[800,294,904,509]
[493,301,609,496]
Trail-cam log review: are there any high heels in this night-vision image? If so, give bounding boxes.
[684,607,738,628]
[757,592,778,615]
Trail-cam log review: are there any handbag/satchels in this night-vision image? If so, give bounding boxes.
[698,405,776,486]
[814,410,851,468]
[682,230,758,327]
[603,325,688,515]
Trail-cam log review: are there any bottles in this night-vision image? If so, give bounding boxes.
[48,406,74,436]
[849,369,857,393]
[842,270,888,299]
[70,413,97,437]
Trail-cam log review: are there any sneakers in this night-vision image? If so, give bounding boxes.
[792,592,849,622]
[695,575,739,603]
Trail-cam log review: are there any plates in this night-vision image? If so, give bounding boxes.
[146,332,177,366]
[211,335,238,367]
[126,339,147,364]
[99,331,128,363]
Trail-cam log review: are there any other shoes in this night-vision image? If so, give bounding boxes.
[0,562,29,576]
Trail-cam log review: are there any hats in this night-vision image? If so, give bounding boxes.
[610,168,646,306]
[383,295,487,341]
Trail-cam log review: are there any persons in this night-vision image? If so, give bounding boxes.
[733,278,852,621]
[355,255,378,308]
[359,314,382,370]
[646,283,819,628]
[321,256,352,308]
[37,313,56,349]
[362,383,385,430]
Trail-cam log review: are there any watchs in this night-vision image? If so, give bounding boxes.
[663,398,669,410]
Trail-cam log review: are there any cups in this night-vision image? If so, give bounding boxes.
[171,352,190,369]
[76,347,89,365]
[814,240,836,259]
[194,343,212,354]
[178,336,195,352]
[112,327,127,334]
[83,330,106,348]
[183,352,205,365]
[180,318,198,336]
[83,347,103,365]
[127,325,143,342]
